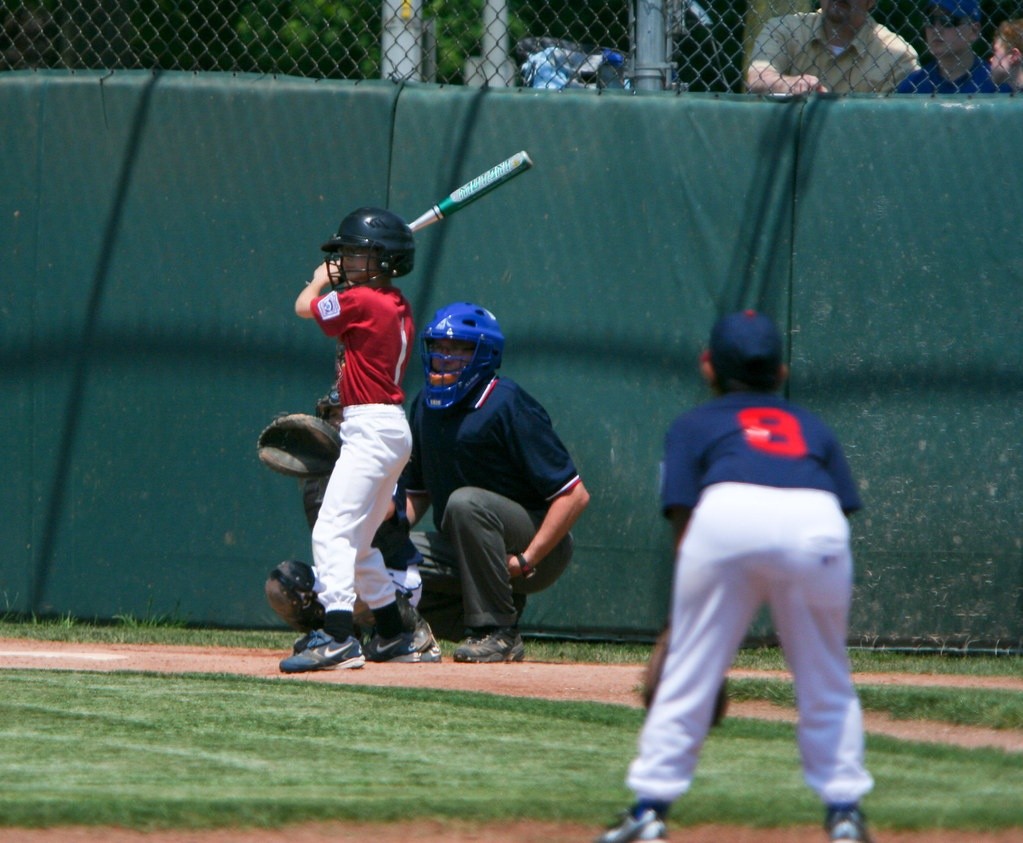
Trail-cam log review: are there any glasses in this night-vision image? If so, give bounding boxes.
[925,15,974,29]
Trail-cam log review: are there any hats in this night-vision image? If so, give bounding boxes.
[924,0,980,21]
[709,310,781,357]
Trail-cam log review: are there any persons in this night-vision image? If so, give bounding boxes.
[383,302,591,662]
[747,0,1023,96]
[263,386,440,658]
[278,209,432,674]
[592,309,876,843]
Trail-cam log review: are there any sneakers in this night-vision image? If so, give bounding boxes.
[825,804,873,842]
[413,618,441,662]
[363,631,420,663]
[602,806,669,843]
[454,626,527,664]
[279,629,365,673]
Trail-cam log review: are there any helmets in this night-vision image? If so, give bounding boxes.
[421,301,505,408]
[321,206,420,289]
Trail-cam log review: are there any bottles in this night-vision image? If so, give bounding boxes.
[595,48,625,89]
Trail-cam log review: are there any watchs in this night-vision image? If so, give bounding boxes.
[516,553,537,579]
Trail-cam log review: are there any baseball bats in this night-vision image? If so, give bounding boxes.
[332,147,533,261]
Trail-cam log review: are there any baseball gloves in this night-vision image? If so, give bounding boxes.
[255,412,343,478]
[640,619,724,724]
